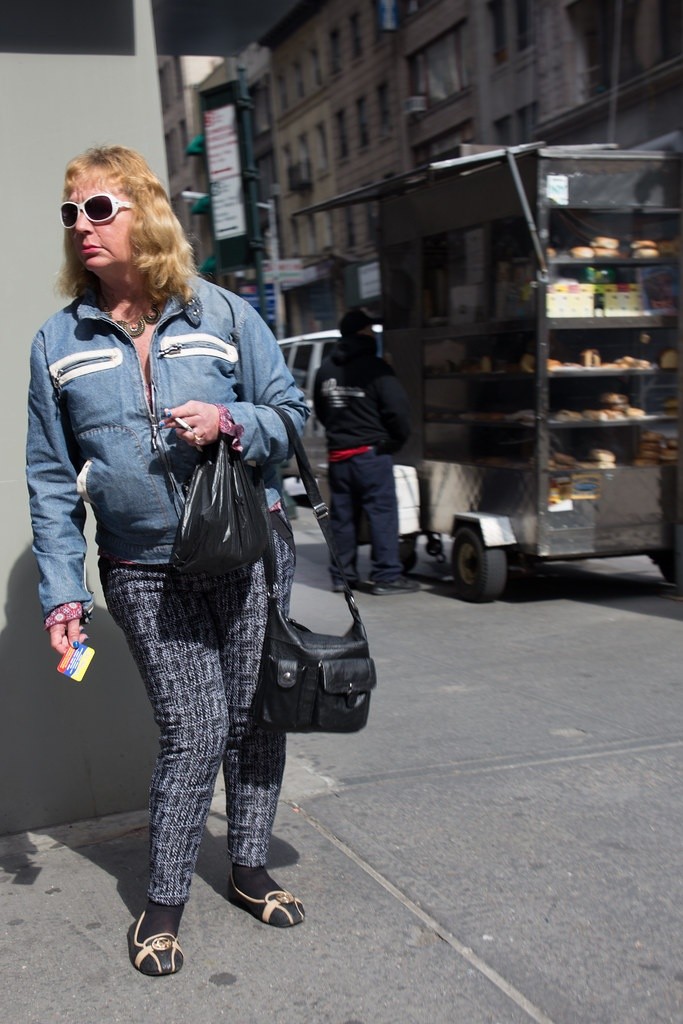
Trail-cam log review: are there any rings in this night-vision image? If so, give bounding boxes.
[195,437,201,445]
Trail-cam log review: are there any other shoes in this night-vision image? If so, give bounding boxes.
[331,571,359,591]
[130,906,183,976]
[373,576,422,597]
[225,868,306,928]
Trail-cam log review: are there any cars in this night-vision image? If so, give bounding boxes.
[274,325,379,482]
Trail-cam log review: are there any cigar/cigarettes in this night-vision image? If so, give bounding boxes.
[164,409,196,430]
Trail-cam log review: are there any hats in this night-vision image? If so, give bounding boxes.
[332,307,386,336]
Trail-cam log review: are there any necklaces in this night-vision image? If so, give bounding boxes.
[99,294,165,340]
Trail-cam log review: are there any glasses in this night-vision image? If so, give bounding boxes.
[59,194,136,228]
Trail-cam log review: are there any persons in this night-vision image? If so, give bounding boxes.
[314,308,421,596]
[23,148,312,977]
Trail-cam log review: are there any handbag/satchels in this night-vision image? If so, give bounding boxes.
[162,413,277,592]
[251,611,375,734]
